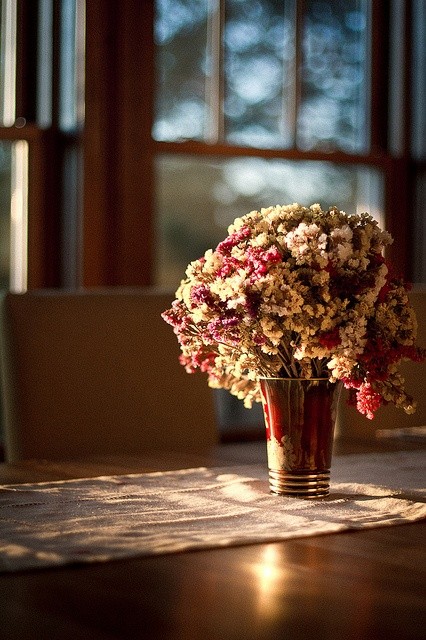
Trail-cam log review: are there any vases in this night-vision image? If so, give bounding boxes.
[257,376,341,498]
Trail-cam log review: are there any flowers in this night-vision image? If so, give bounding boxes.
[158,201,424,419]
